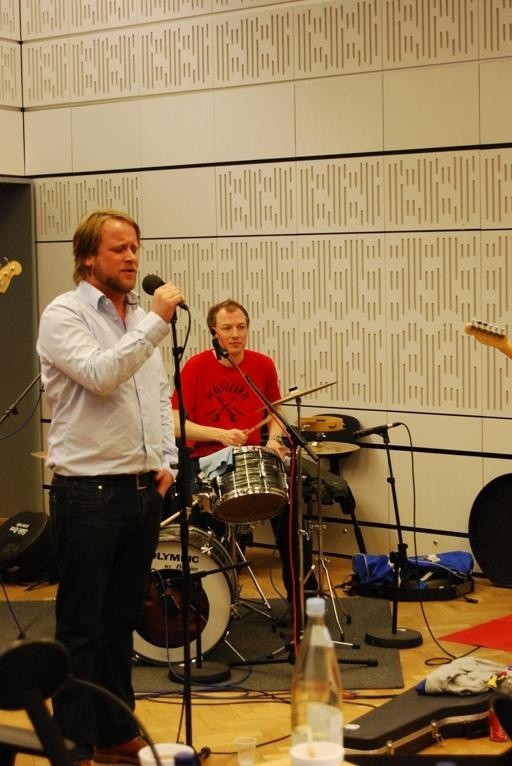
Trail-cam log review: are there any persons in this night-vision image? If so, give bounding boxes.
[35,209,179,766]
[171,298,318,631]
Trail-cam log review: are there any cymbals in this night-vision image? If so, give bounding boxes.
[257,381,336,412]
[279,442,360,455]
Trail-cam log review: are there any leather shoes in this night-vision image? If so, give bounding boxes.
[90,735,151,765]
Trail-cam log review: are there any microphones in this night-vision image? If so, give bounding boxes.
[212,331,223,359]
[354,422,400,437]
[142,274,185,310]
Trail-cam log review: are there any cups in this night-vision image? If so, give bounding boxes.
[137,742,196,766]
[236,738,257,766]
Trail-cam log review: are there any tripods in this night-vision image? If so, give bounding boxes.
[231,501,380,671]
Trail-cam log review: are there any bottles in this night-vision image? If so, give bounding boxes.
[289,598,347,766]
[488,664,511,742]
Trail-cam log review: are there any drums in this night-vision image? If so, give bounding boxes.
[298,416,344,431]
[133,525,240,662]
[209,446,288,526]
